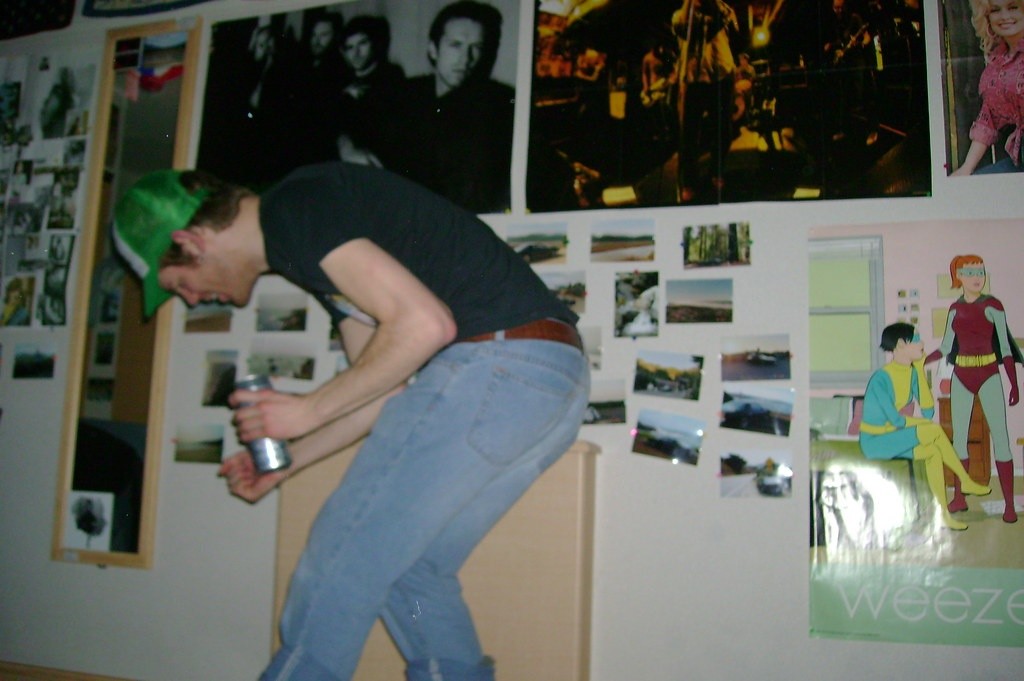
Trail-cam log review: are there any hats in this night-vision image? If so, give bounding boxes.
[112,168,225,318]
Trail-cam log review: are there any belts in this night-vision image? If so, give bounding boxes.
[451,319,582,351]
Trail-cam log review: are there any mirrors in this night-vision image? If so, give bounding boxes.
[47,14,205,571]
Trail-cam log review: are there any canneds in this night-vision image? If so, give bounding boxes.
[233,374,292,472]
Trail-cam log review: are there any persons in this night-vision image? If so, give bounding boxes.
[536,0,755,124]
[824,0,873,64]
[247,1,515,213]
[112,161,593,681]
[947,0,1024,178]
